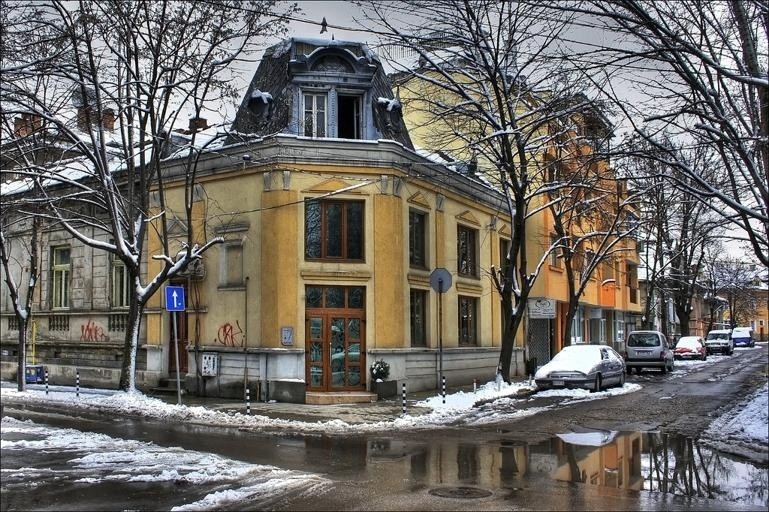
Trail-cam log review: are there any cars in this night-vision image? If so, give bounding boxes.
[535,345,625,390]
[673,335,706,359]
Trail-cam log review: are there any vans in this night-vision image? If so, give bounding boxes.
[624,329,674,373]
[706,325,755,355]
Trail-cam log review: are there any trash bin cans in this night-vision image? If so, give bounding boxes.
[527,357,537,377]
